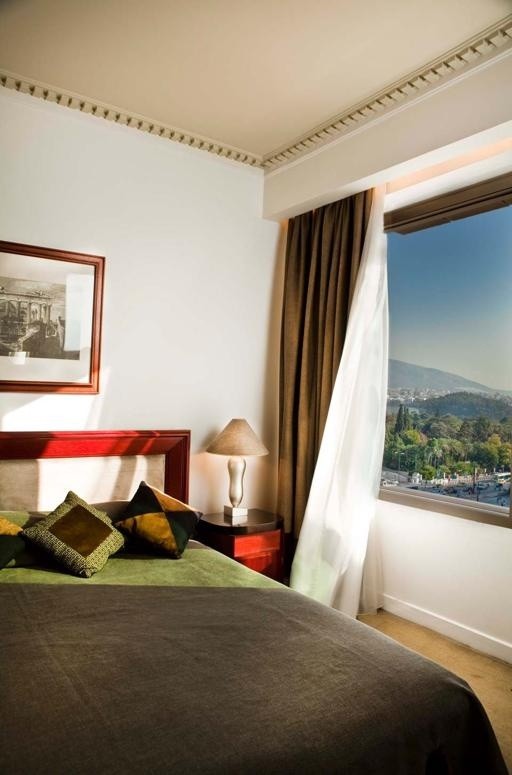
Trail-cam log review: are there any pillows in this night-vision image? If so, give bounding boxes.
[0,480,203,576]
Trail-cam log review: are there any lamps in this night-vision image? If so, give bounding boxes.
[206,418,270,517]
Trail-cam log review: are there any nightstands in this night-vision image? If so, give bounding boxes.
[197,508,285,580]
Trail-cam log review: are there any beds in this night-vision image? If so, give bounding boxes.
[0,430,509,775]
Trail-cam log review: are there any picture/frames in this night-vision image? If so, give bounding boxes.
[0,239,106,394]
